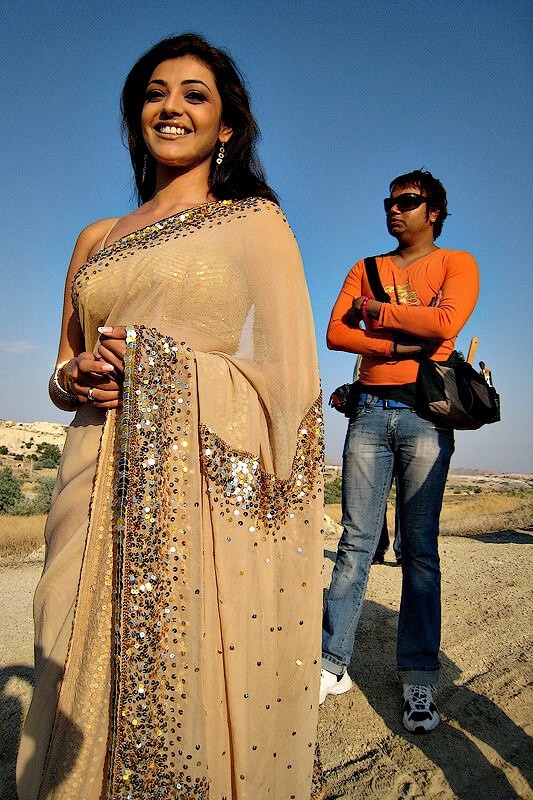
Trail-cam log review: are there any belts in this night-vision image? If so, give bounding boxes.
[358,394,412,408]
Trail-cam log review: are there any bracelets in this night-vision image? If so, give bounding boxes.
[53,359,78,400]
[361,295,375,321]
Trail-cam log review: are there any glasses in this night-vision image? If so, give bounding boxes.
[384,193,430,213]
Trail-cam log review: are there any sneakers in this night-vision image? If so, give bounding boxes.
[318,668,353,704]
[400,683,441,733]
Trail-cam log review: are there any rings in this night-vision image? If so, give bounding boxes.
[88,387,94,401]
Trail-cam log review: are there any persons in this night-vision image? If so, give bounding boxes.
[16,34,324,800]
[317,170,481,733]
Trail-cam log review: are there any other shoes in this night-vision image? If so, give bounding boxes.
[374,556,384,564]
[397,558,402,567]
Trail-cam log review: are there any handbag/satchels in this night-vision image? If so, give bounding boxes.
[416,349,493,430]
